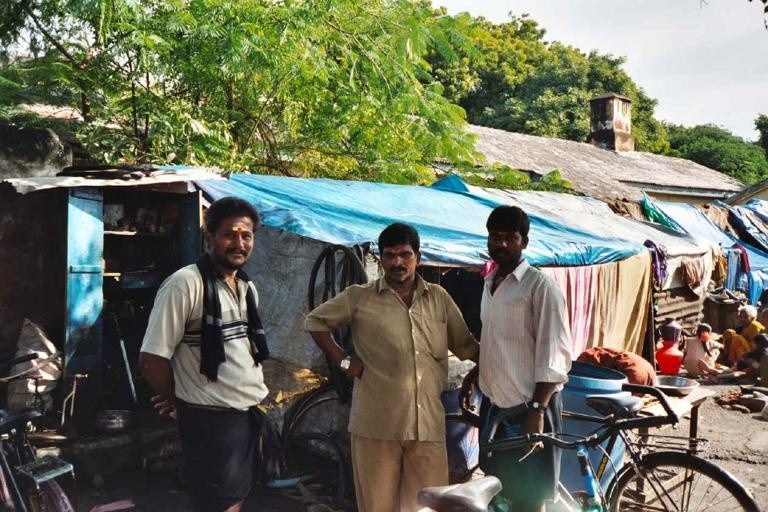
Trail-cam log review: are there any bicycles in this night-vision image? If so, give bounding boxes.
[1,350,74,512]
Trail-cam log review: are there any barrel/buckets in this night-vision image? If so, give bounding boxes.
[54,410,144,486]
[558,359,630,499]
[440,384,483,479]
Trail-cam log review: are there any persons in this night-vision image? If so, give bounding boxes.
[684,288,768,385]
[137,197,270,512]
[302,224,479,512]
[458,206,572,512]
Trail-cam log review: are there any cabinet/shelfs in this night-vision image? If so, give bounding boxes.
[105,230,183,323]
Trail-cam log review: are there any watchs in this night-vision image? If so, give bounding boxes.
[527,401,546,410]
[340,355,352,375]
